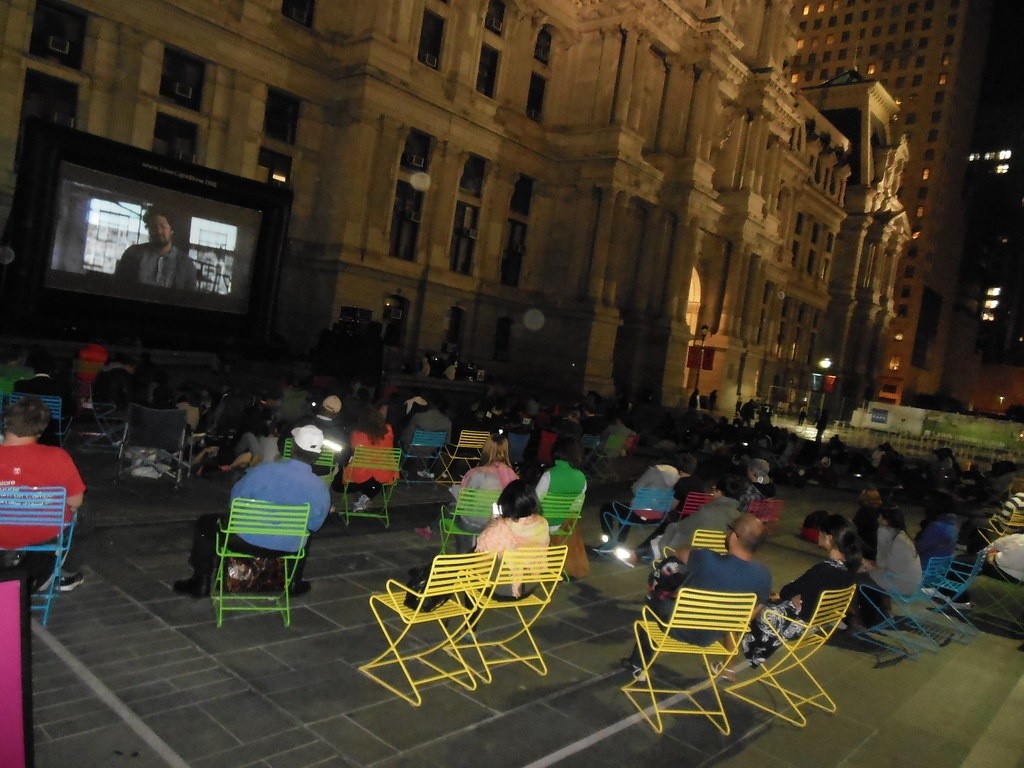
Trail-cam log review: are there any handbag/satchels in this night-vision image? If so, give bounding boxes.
[647,556,689,611]
[404,564,454,613]
[549,519,591,576]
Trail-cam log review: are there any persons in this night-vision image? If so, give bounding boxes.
[615,514,771,680]
[0,343,1024,608]
[116,206,196,289]
[712,512,864,683]
[828,504,922,634]
[460,478,551,641]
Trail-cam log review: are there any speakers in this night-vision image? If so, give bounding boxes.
[384,323,400,346]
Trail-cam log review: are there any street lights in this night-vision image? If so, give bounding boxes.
[814,358,831,426]
[694,325,709,390]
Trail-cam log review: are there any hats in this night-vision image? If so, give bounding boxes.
[323,395,341,413]
[290,424,324,453]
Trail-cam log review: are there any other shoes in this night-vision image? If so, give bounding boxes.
[593,543,615,553]
[458,628,478,644]
[415,528,432,541]
[285,579,311,597]
[417,470,435,479]
[173,580,210,599]
[53,571,84,593]
[621,658,652,677]
[441,471,448,479]
[705,661,736,681]
[352,495,370,512]
[33,564,56,592]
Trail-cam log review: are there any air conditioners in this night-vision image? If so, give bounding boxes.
[486,18,503,32]
[514,245,526,255]
[529,110,540,122]
[421,53,437,68]
[176,151,196,164]
[408,154,424,167]
[408,211,423,224]
[175,82,192,99]
[48,35,70,55]
[467,228,480,241]
[48,113,75,129]
[444,342,457,352]
[384,308,402,319]
[288,7,307,24]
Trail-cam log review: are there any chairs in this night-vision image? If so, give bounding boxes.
[0,486,77,626]
[111,402,191,492]
[206,498,312,629]
[723,584,855,729]
[970,576,1024,637]
[442,545,569,684]
[854,553,954,659]
[440,486,504,553]
[334,444,402,530]
[977,507,1024,545]
[281,438,337,487]
[77,383,123,451]
[358,549,499,707]
[904,547,990,643]
[9,391,73,447]
[539,492,585,548]
[620,587,757,737]
[579,433,640,481]
[435,430,491,486]
[597,486,783,571]
[397,427,446,483]
